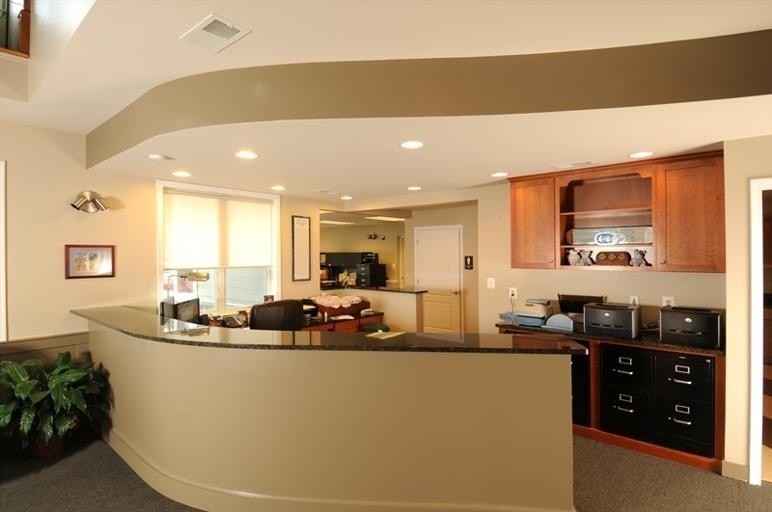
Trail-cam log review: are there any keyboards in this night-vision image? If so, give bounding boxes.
[223,317,242,328]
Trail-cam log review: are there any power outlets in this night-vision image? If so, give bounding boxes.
[509,288,517,299]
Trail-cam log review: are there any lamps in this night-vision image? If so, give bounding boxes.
[164,273,210,297]
[70,190,110,213]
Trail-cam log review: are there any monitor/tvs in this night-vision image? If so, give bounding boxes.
[160,296,175,315]
[176,297,200,326]
[250,300,304,330]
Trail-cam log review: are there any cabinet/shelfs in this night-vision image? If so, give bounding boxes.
[506,148,723,274]
[497,323,725,475]
[304,311,384,332]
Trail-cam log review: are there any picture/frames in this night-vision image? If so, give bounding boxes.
[292,215,311,281]
[65,245,116,279]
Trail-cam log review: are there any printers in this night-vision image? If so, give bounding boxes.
[660,305,723,350]
[583,302,642,340]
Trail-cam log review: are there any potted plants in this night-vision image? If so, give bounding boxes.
[0,350,114,460]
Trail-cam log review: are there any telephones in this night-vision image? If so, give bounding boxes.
[222,314,241,328]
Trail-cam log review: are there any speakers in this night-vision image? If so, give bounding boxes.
[200,314,209,325]
